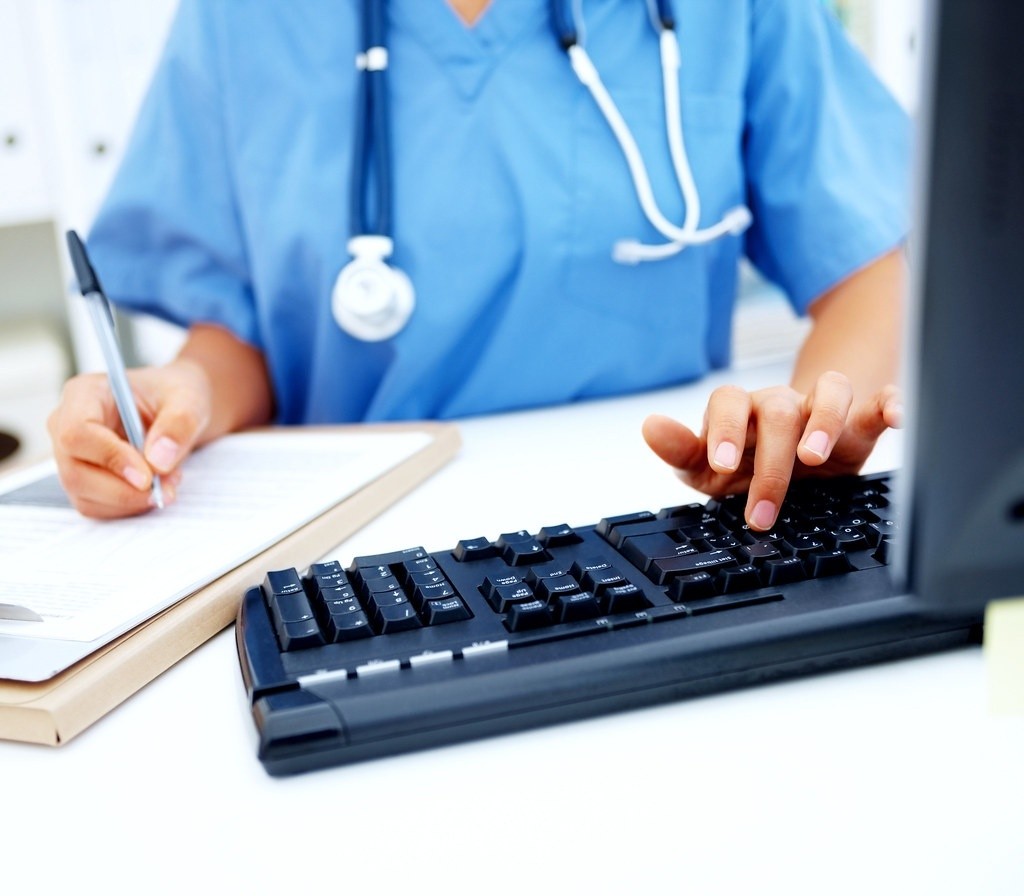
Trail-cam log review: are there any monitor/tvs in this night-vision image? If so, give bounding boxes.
[890,0,1024,604]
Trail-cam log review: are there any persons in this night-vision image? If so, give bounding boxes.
[47,0,915,533]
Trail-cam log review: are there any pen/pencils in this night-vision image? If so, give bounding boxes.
[66,230,165,512]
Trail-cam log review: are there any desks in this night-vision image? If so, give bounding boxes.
[0,278,1024,896]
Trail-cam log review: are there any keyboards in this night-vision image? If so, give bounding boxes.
[227,466,983,778]
